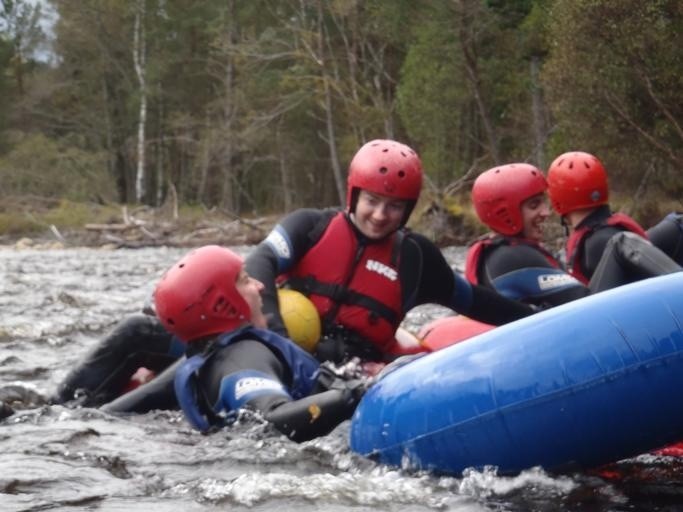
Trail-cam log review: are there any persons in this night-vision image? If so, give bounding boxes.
[151,242,373,451]
[545,149,651,286]
[641,212,683,267]
[463,162,683,320]
[0,135,546,420]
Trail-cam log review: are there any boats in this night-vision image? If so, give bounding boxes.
[349,270,683,480]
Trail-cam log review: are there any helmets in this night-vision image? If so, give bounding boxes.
[347,138,422,227]
[155,242,253,346]
[547,150,611,217]
[471,162,548,235]
